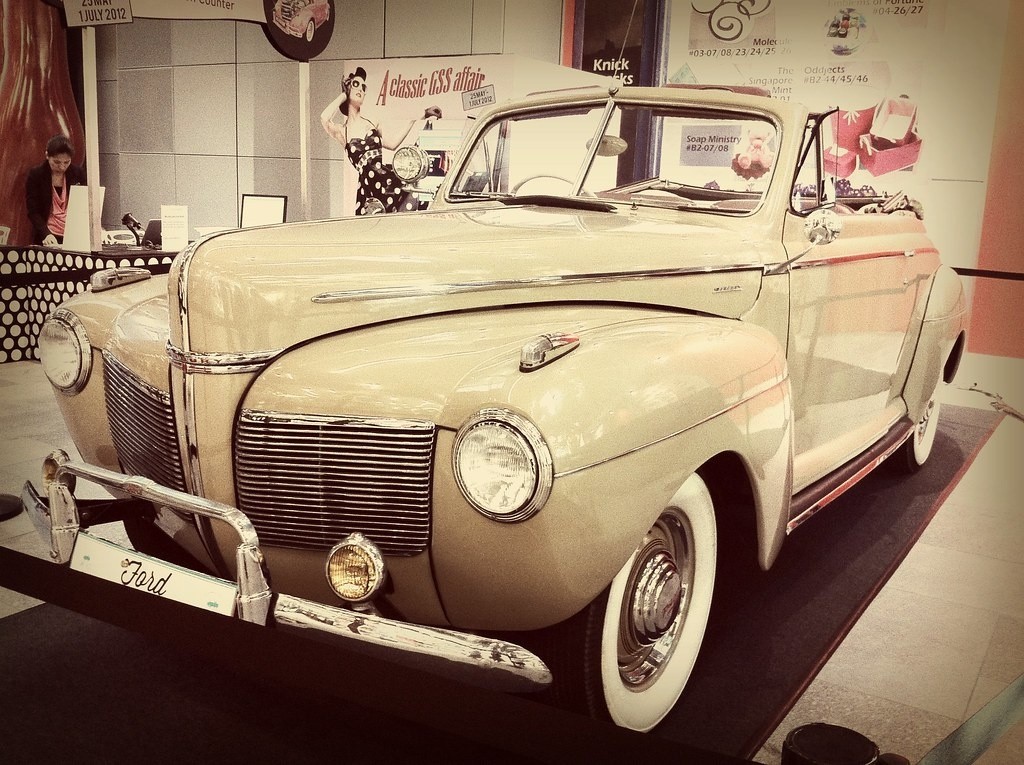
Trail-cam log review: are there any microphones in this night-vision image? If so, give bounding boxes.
[121,212,141,230]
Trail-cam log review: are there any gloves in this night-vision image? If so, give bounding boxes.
[425,106,442,120]
[341,75,351,99]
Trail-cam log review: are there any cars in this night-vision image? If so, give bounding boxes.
[273,0,331,42]
[0,82,971,749]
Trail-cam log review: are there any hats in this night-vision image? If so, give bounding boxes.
[339,67,366,115]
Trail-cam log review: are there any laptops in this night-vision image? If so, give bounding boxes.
[140,219,162,247]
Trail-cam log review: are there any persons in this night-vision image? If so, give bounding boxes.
[25,133,88,246]
[318,66,443,217]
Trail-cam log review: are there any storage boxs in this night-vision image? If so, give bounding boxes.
[824,95,922,178]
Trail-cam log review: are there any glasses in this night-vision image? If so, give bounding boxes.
[352,80,367,92]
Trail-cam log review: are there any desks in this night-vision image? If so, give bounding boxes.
[0,238,195,362]
